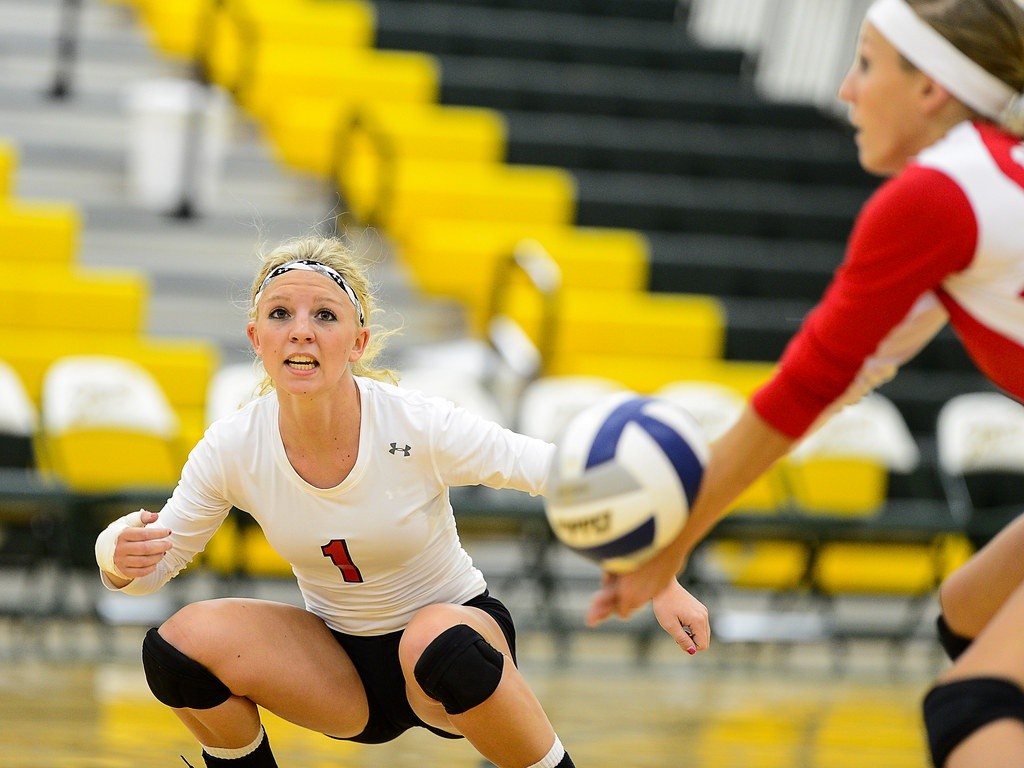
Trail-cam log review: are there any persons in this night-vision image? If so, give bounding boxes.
[93,236,713,768]
[584,2,1024,768]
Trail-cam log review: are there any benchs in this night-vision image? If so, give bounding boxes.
[0,0,1001,634]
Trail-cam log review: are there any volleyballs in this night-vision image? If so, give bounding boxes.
[543,391,714,570]
